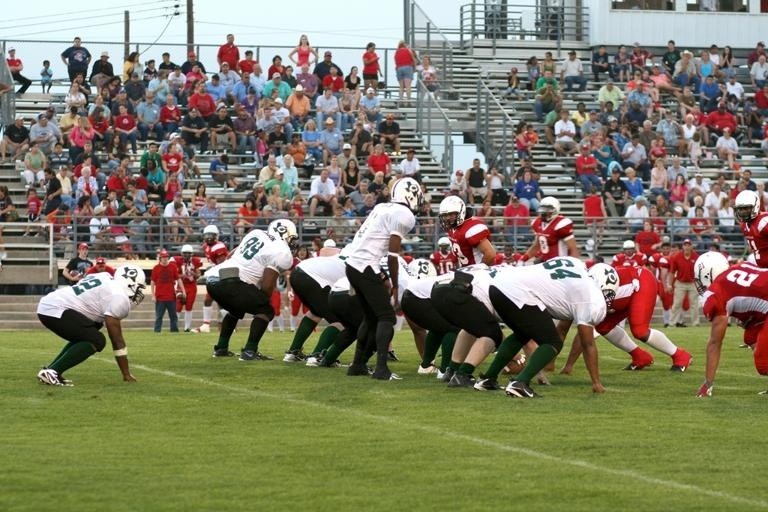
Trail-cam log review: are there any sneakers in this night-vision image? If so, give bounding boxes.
[37,367,74,387]
[192,324,210,333]
[416,344,541,398]
[238,347,272,361]
[621,351,655,370]
[211,344,241,357]
[60,374,72,383]
[677,322,687,327]
[671,352,694,373]
[283,346,407,383]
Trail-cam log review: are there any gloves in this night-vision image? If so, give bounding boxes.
[694,382,713,398]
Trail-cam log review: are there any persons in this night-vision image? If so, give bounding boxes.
[0,34,768,398]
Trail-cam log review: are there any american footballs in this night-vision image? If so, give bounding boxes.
[502,354,527,375]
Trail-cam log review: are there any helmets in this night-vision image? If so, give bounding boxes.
[588,263,620,313]
[536,196,561,223]
[114,263,148,306]
[181,245,194,261]
[692,251,730,298]
[390,176,467,279]
[267,219,299,250]
[623,240,635,257]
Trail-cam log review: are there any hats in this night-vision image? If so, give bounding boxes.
[202,225,219,246]
[510,195,519,204]
[159,251,169,257]
[272,72,393,128]
[684,239,692,246]
[560,42,764,150]
[14,106,77,122]
[94,205,106,215]
[342,143,351,150]
[275,168,283,176]
[118,72,156,98]
[101,52,110,58]
[723,127,731,133]
[613,157,704,214]
[732,190,760,223]
[79,243,88,249]
[176,191,182,197]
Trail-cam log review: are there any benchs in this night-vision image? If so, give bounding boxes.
[468,54,767,222]
[1,67,453,216]
